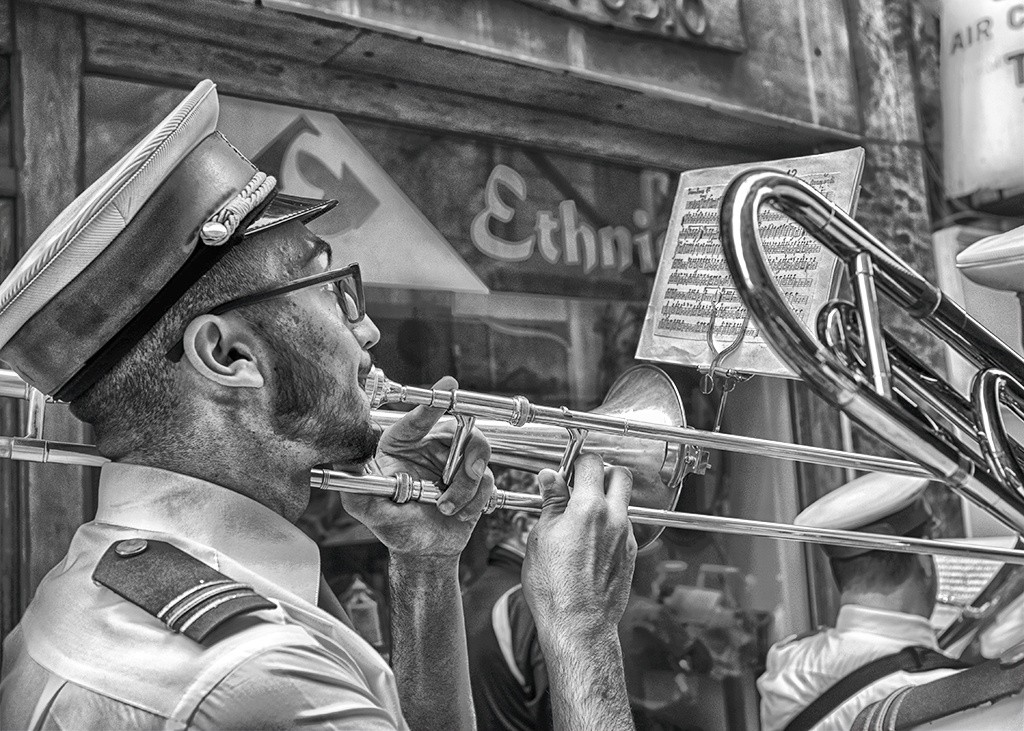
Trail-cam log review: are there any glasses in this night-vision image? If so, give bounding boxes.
[165,263,370,366]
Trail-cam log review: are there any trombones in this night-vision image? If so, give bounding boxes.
[0,369,1024,562]
[723,164,1019,547]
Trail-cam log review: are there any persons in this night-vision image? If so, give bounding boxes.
[753,460,1024,731]
[1,72,641,731]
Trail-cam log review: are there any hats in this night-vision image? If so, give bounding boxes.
[0,78,337,410]
[787,461,952,557]
